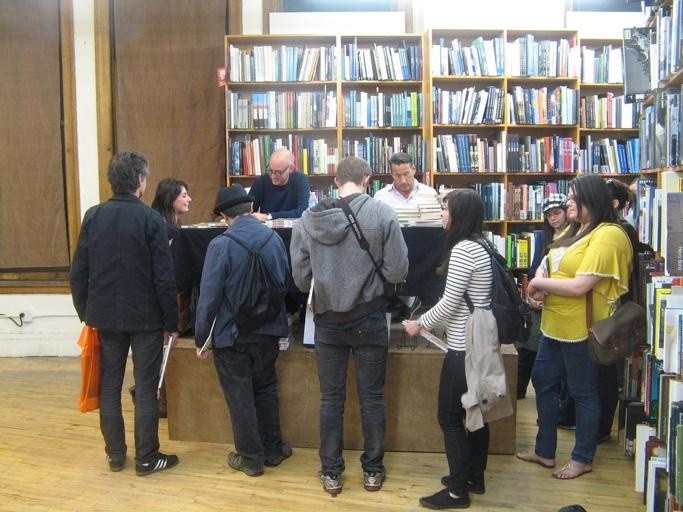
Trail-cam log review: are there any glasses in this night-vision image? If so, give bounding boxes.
[267,166,290,176]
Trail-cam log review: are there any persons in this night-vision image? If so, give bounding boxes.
[70,150,178,476]
[405,189,501,510]
[516,168,639,477]
[194,183,290,478]
[375,152,444,227]
[129,178,192,418]
[248,149,309,219]
[292,155,408,493]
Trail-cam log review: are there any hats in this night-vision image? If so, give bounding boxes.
[544,193,566,211]
[213,183,255,214]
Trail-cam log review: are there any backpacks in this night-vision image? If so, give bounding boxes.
[221,230,286,335]
[463,237,531,344]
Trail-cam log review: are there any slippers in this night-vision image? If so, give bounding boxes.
[553,461,592,480]
[516,451,554,467]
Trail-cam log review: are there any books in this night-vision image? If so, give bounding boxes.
[615,4,682,510]
[157,335,172,391]
[199,317,217,357]
[433,34,641,300]
[227,40,423,210]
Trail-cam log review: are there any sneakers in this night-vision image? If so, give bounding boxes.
[598,433,611,444]
[442,476,484,492]
[320,472,343,494]
[135,453,177,477]
[421,490,470,509]
[536,418,576,430]
[363,470,386,490]
[394,296,420,323]
[108,455,122,472]
[266,445,291,466]
[228,452,263,476]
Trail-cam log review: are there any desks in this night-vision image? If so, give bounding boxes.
[175,220,451,332]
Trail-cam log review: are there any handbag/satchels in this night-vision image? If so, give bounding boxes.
[587,301,647,367]
